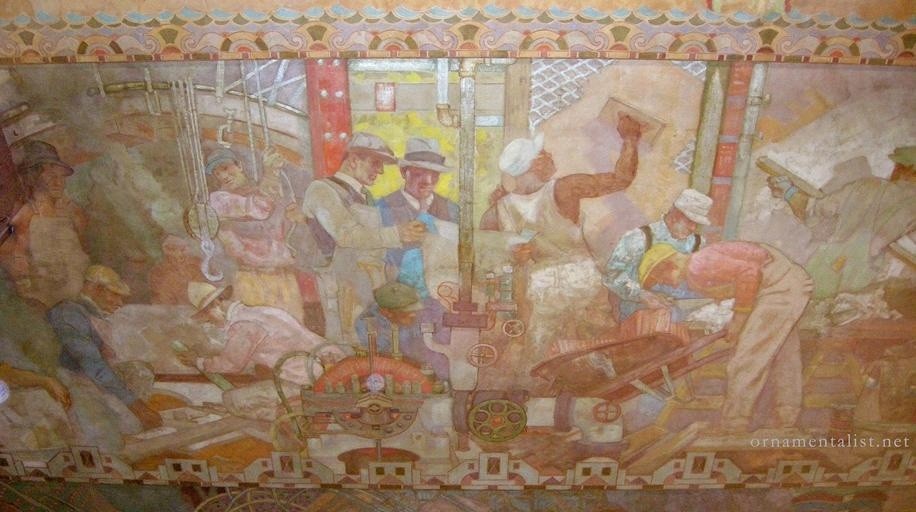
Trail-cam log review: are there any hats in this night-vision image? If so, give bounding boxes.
[397,137,450,173]
[887,146,916,167]
[84,264,132,297]
[206,149,235,175]
[638,242,677,290]
[18,140,74,176]
[675,188,715,225]
[498,134,547,179]
[373,283,427,313]
[346,133,396,165]
[188,281,234,323]
